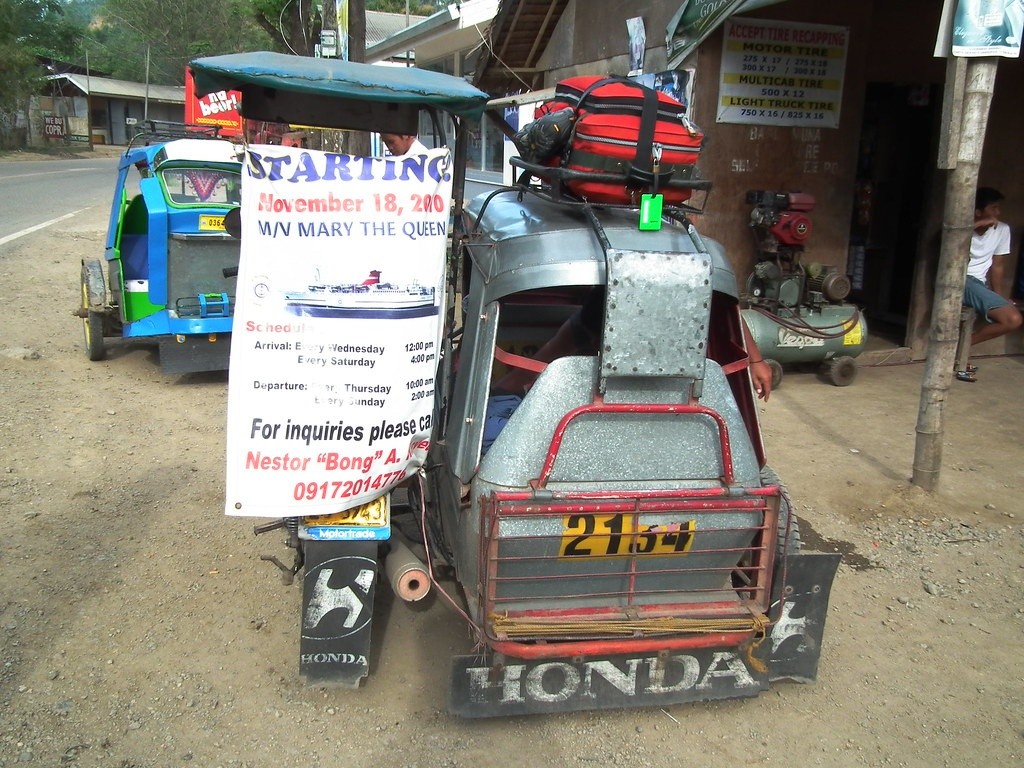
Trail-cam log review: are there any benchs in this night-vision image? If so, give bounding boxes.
[116,232,166,321]
[951,306,1024,376]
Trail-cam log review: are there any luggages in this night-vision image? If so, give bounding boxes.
[530,76,703,202]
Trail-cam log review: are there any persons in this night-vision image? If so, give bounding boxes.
[954,184,1022,372]
[491,286,772,403]
[381,134,430,156]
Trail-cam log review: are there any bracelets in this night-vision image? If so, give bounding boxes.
[748,360,764,364]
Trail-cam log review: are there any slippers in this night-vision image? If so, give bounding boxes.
[954,363,978,370]
[956,371,977,381]
